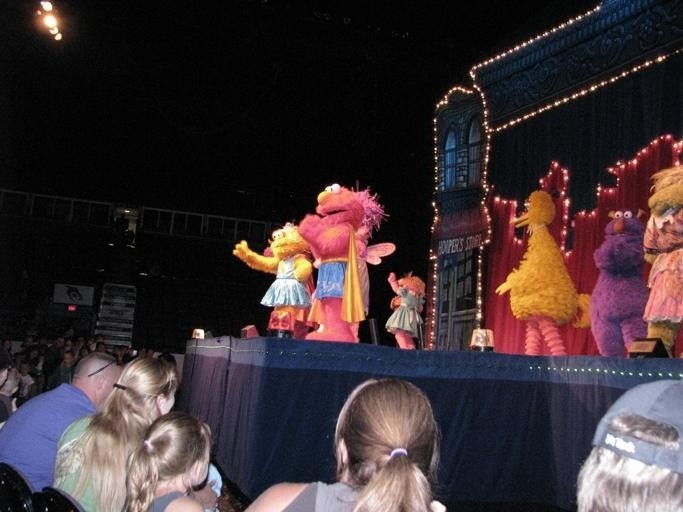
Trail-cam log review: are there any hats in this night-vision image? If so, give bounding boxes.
[591,380,682,475]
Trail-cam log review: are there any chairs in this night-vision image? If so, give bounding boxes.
[0,463,32,512]
[32,487,85,512]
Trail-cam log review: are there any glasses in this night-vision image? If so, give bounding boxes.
[88,358,120,377]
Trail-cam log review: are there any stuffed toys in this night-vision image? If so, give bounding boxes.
[641,167,683,359]
[494,190,592,358]
[385,270,427,351]
[589,208,649,359]
[230,180,391,344]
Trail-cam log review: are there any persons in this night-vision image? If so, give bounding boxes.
[574,378,683,512]
[244,377,447,512]
[0,337,242,512]
